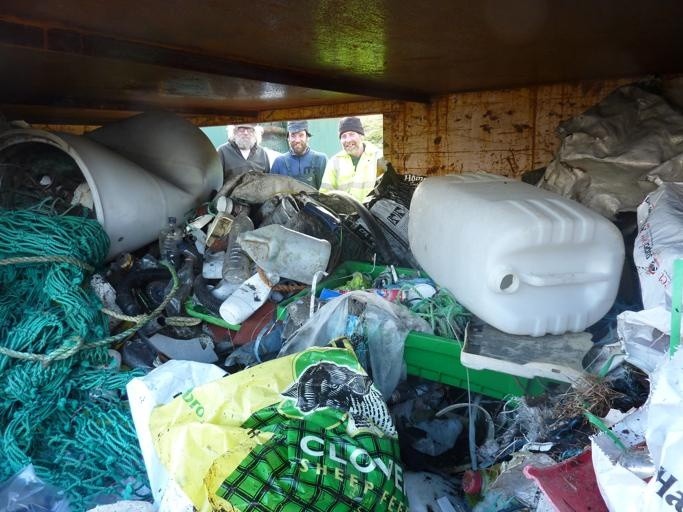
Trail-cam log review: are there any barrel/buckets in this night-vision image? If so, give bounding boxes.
[407,168,626,339]
[235,223,333,287]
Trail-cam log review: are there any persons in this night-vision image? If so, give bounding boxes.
[217,124,270,187]
[318,118,382,206]
[270,120,326,190]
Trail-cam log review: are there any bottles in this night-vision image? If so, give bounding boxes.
[117,194,280,326]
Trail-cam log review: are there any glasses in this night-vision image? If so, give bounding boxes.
[238,127,255,132]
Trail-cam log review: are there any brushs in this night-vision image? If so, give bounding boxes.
[462,396,482,495]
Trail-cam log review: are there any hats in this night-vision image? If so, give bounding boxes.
[286,121,312,138]
[339,117,364,140]
